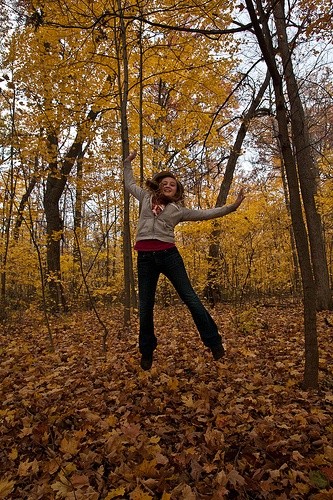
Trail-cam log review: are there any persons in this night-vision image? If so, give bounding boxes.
[122,149,247,371]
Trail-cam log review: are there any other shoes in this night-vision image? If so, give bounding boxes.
[140,351,153,370]
[209,342,224,359]
